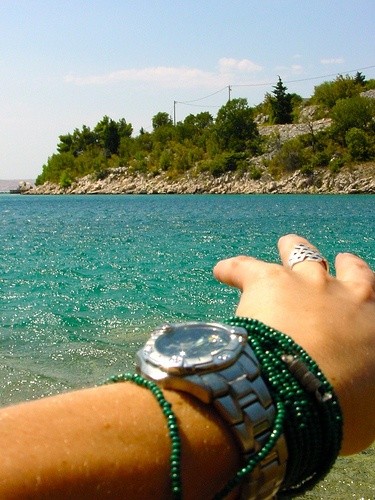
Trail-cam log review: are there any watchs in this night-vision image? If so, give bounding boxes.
[137,322,285,500]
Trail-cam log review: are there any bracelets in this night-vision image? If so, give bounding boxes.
[99,317,345,500]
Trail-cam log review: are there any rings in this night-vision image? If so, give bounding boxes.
[286,242,330,272]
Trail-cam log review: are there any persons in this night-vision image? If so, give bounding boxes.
[2,235,375,499]
